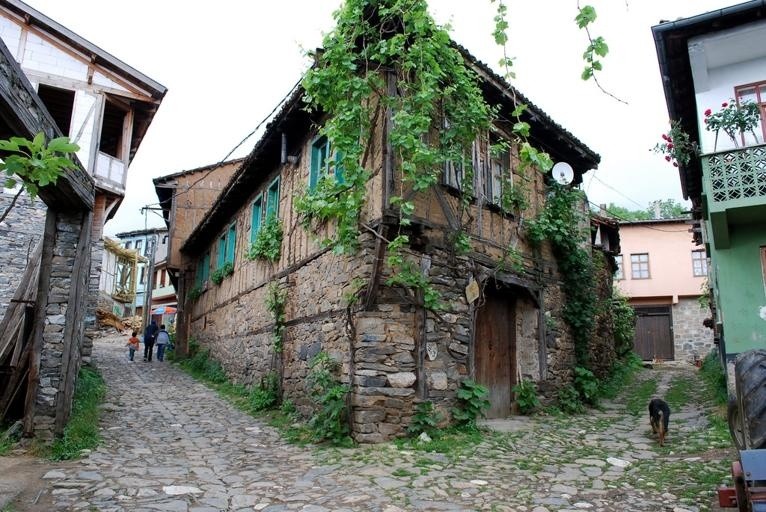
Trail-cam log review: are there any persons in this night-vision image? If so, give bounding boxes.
[123,331,143,362]
[143,319,158,363]
[154,324,171,363]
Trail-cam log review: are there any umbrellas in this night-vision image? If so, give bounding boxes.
[148,305,179,324]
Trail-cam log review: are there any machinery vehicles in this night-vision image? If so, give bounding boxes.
[716,347,766,512]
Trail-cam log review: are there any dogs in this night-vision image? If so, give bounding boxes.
[648,398,669,446]
